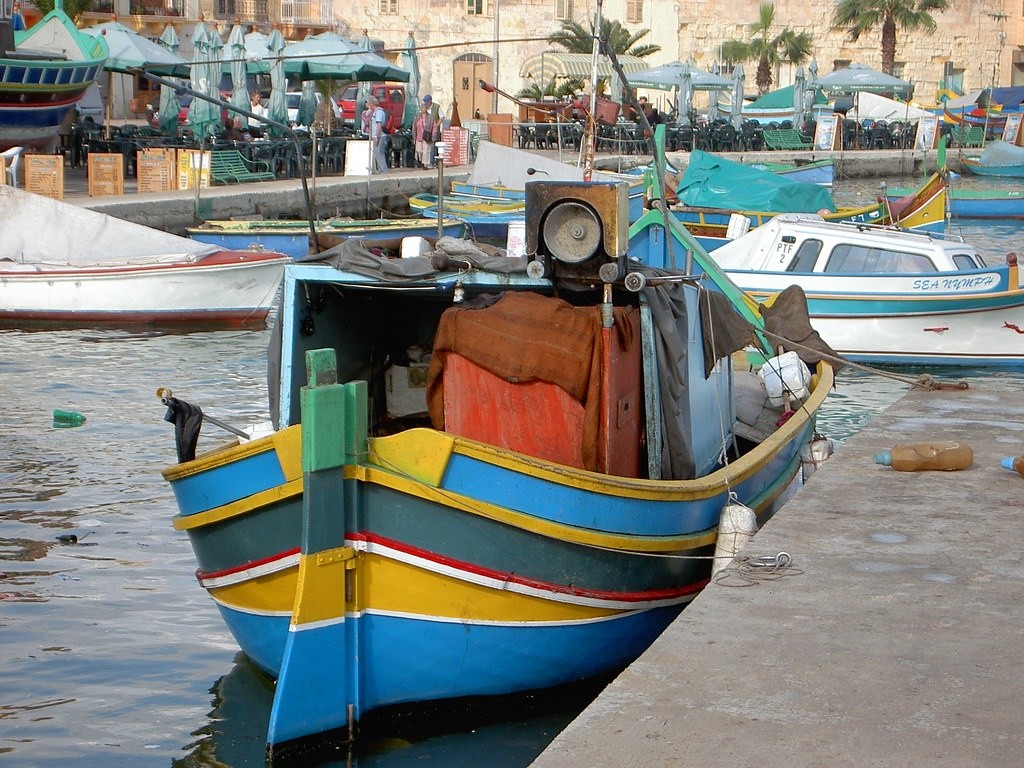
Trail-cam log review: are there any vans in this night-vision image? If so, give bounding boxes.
[334,81,406,128]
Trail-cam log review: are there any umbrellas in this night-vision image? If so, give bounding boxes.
[625,57,915,132]
[78,14,421,141]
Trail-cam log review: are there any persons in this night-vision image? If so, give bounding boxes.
[222,92,445,173]
[629,96,647,119]
[647,108,662,124]
[475,108,480,119]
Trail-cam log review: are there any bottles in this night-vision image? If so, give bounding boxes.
[872,443,974,471]
[1000,454,1024,477]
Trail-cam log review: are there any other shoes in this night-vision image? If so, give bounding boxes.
[423,166,427,170]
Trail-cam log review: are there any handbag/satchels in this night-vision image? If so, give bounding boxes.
[422,129,432,142]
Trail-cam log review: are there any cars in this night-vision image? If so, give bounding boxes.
[148,92,233,135]
[259,92,343,134]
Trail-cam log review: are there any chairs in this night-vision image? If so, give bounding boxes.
[4,151,21,188]
[55,125,413,177]
[517,115,986,154]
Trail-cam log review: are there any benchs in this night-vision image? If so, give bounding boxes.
[761,129,815,150]
[208,150,276,185]
[951,127,989,149]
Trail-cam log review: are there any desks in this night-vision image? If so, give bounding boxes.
[519,122,576,152]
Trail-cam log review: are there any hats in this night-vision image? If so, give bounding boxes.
[423,95,432,102]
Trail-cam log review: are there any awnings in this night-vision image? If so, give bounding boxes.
[519,52,651,93]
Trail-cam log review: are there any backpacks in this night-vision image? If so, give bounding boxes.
[376,109,396,135]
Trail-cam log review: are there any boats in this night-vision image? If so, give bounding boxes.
[0,181,295,330]
[109,1,838,747]
[183,76,1024,269]
[704,212,1023,372]
